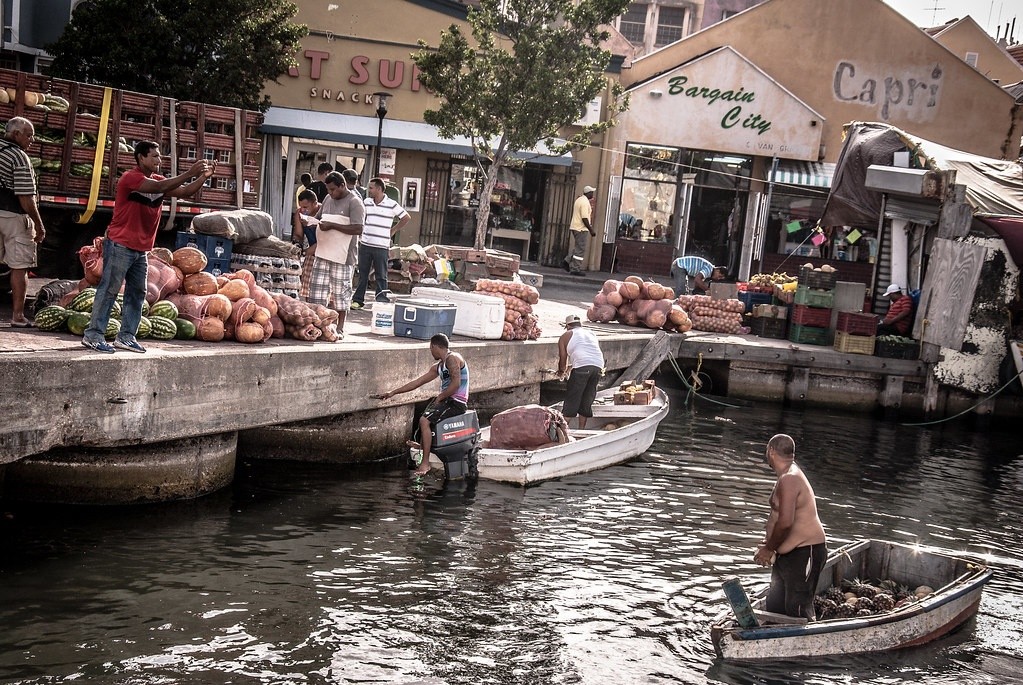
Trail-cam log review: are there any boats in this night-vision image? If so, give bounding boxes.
[407,379,672,487]
[707,538,997,663]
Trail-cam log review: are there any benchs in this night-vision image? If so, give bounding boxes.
[570,427,612,438]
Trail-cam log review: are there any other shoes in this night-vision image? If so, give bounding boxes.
[571,270,584,276]
[560,260,569,273]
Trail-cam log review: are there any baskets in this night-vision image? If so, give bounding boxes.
[875,339,920,360]
[738,265,837,346]
[836,310,878,337]
[834,332,875,355]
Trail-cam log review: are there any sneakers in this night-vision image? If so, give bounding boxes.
[81,337,116,353]
[350,302,364,310]
[114,339,146,353]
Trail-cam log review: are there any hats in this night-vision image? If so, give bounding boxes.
[583,186,596,194]
[564,315,580,329]
[882,284,901,296]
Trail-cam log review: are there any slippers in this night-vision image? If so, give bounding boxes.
[11,320,36,328]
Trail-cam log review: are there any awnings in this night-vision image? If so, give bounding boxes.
[766,160,836,188]
[258,107,572,166]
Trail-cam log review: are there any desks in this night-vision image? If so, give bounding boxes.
[487,227,532,260]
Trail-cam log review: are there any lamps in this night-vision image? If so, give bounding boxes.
[649,89,663,98]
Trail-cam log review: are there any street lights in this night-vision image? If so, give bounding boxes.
[372,91,394,176]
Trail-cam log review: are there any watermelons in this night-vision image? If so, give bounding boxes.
[34,287,196,342]
[0,95,137,181]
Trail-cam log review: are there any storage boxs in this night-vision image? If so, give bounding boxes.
[734,268,918,361]
[487,255,519,270]
[176,230,232,260]
[490,267,514,277]
[411,286,505,340]
[613,390,631,404]
[204,259,230,275]
[387,247,401,260]
[519,269,546,286]
[394,297,458,339]
[620,380,634,390]
[634,389,651,404]
[643,379,656,398]
[485,248,521,261]
[437,245,486,262]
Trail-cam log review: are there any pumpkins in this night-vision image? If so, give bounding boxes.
[62,240,285,343]
[0,87,46,105]
[586,276,693,333]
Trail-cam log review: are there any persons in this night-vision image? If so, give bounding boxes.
[81,141,217,354]
[292,190,322,301]
[561,186,597,276]
[671,257,728,299]
[296,162,366,207]
[380,333,469,474]
[301,171,366,341]
[620,213,643,232]
[753,434,828,621]
[0,117,46,327]
[557,314,605,429]
[876,284,912,336]
[350,177,411,308]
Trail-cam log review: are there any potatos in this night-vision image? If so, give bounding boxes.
[675,294,748,335]
[269,294,341,341]
[475,279,541,341]
